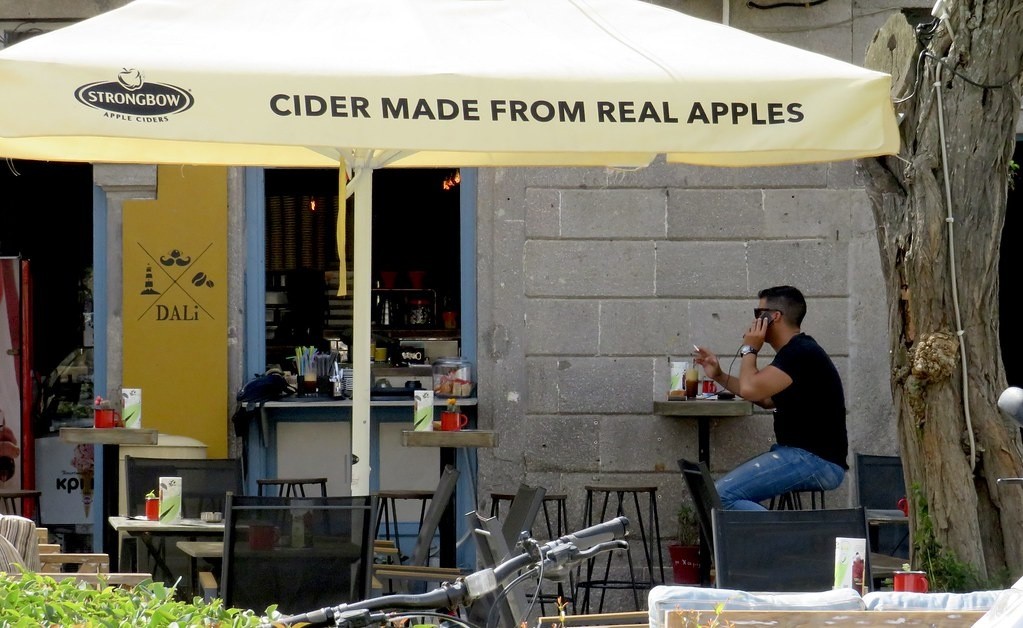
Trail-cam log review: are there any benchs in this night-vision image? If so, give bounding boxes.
[6,528,152,587]
[648,586,1007,628]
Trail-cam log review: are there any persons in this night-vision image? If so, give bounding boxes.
[693,285,849,510]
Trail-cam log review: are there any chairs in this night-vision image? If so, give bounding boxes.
[124,455,372,614]
[853,453,910,590]
[678,459,874,596]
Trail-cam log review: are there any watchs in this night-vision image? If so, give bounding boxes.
[740,345,757,357]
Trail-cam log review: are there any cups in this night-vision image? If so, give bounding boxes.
[146,497,159,521]
[94,407,121,428]
[410,271,425,288]
[303,368,317,397]
[383,272,397,288]
[702,380,717,393]
[897,498,908,517]
[685,369,698,397]
[371,343,376,357]
[442,311,458,329]
[375,348,387,361]
[440,411,468,431]
[892,570,928,593]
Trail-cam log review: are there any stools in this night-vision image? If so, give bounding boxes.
[769,486,826,511]
[0,489,42,528]
[258,479,665,624]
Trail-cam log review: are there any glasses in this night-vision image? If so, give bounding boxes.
[754,307,783,319]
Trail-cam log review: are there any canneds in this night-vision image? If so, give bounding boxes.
[371,344,388,366]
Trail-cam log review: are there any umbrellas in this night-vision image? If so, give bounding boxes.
[0,0,903,604]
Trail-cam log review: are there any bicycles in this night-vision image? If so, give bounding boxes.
[274,516,629,628]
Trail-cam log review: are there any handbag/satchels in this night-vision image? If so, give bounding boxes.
[237,374,286,398]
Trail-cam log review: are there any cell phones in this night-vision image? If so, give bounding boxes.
[743,311,775,338]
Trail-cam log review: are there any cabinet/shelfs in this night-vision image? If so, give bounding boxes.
[52,375,94,430]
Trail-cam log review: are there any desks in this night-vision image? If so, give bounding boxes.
[175,541,361,603]
[403,430,499,573]
[653,401,754,471]
[110,516,280,602]
[59,428,159,587]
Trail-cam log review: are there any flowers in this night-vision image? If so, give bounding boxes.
[95,395,110,408]
[447,398,460,412]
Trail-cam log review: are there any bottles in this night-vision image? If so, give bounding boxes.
[432,356,473,398]
[407,299,430,326]
[375,276,383,289]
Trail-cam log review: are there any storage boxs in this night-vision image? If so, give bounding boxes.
[159,477,182,523]
[414,390,434,432]
[121,388,141,429]
[671,361,689,391]
[835,537,866,595]
[291,509,312,548]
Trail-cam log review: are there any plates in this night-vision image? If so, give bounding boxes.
[342,369,353,391]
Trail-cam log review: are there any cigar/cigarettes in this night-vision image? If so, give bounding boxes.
[694,344,699,351]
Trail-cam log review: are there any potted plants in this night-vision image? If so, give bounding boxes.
[667,501,703,584]
